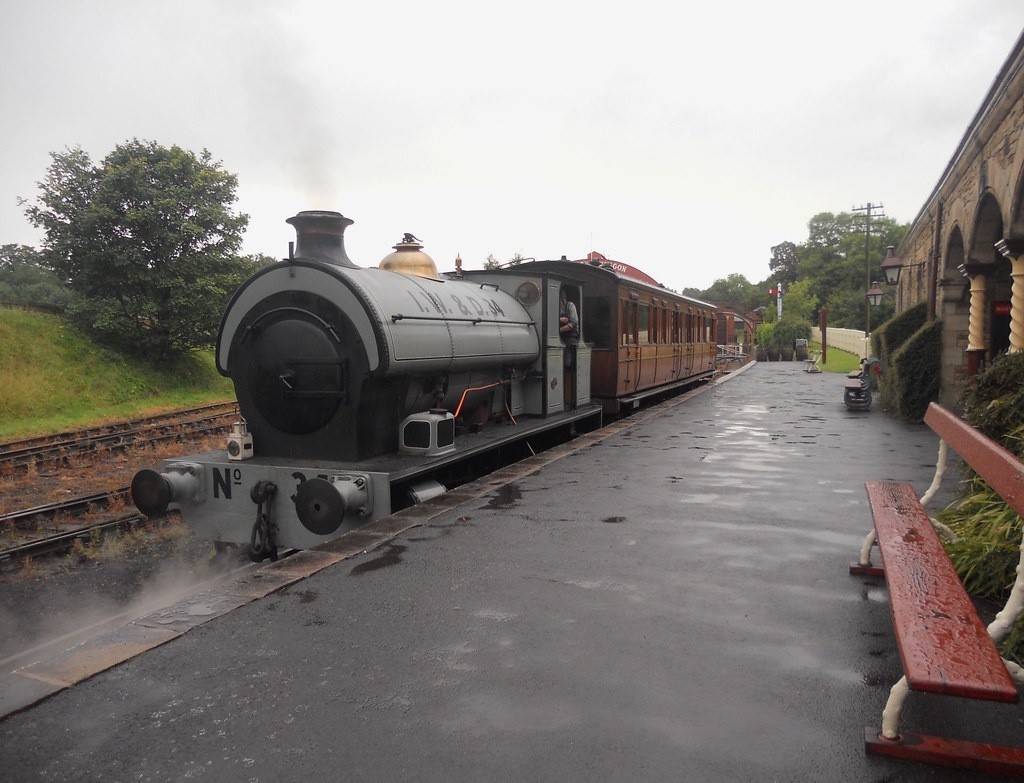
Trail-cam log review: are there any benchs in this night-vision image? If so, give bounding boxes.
[803,349,817,371]
[849,397,1024,781]
[844,359,872,410]
[845,357,868,380]
[807,350,823,373]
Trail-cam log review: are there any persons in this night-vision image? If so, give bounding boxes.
[559,288,579,370]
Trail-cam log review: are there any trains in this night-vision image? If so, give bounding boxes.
[129,210,735,559]
